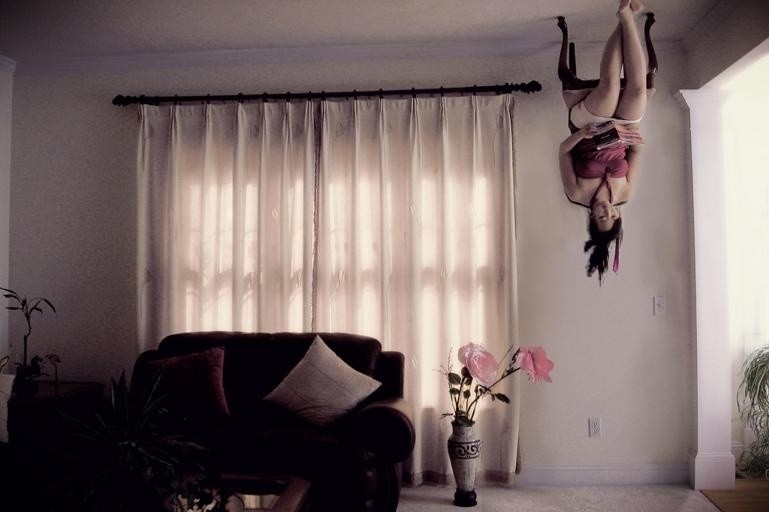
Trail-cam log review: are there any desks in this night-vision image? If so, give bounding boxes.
[695,487,768,511]
[86,476,314,512]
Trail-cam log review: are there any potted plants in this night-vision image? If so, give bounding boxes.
[0,285,61,397]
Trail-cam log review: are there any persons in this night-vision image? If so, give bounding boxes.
[558,0,644,287]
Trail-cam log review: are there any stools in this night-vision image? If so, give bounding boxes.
[555,10,660,111]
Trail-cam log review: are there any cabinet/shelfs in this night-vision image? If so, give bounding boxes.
[6,379,113,449]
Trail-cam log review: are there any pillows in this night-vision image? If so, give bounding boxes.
[264,333,385,432]
[145,344,234,420]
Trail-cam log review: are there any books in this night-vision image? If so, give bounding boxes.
[592,121,643,150]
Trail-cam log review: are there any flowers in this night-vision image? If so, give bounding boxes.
[433,340,555,425]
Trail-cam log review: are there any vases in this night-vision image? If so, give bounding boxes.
[446,420,483,491]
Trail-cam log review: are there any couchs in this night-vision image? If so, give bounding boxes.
[131,329,418,512]
